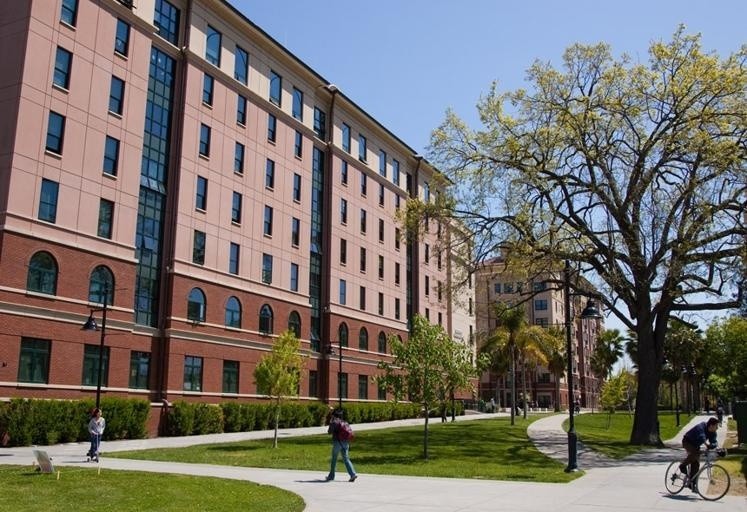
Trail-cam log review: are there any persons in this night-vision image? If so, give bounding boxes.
[323,405,358,482]
[490,396,496,413]
[86,406,106,463]
[715,396,724,428]
[678,417,718,492]
[703,396,711,415]
[574,397,580,416]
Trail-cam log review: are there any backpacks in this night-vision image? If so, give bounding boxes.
[333,420,355,441]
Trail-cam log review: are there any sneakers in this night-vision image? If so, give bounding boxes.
[326,477,333,480]
[348,475,357,482]
[679,464,687,474]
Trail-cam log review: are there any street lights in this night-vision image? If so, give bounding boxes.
[320,328,346,415]
[558,256,603,474]
[75,278,111,455]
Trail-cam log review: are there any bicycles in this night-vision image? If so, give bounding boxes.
[665,442,732,501]
